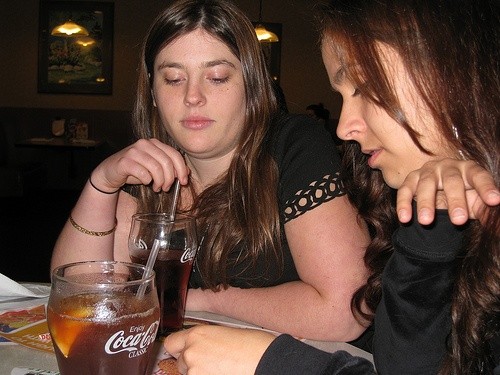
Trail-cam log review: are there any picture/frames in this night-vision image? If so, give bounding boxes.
[37,0,114,94]
[251,21,283,84]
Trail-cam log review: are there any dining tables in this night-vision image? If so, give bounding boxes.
[15,139,108,215]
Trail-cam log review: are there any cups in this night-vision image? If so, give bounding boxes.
[128,212,198,343]
[47,259,160,375]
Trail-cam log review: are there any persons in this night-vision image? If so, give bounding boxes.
[163,0,500,375]
[51,0,375,343]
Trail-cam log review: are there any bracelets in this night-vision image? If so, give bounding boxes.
[69,213,119,237]
[89,176,121,195]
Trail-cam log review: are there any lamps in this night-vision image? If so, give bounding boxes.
[51,0,88,37]
[253,0,278,44]
